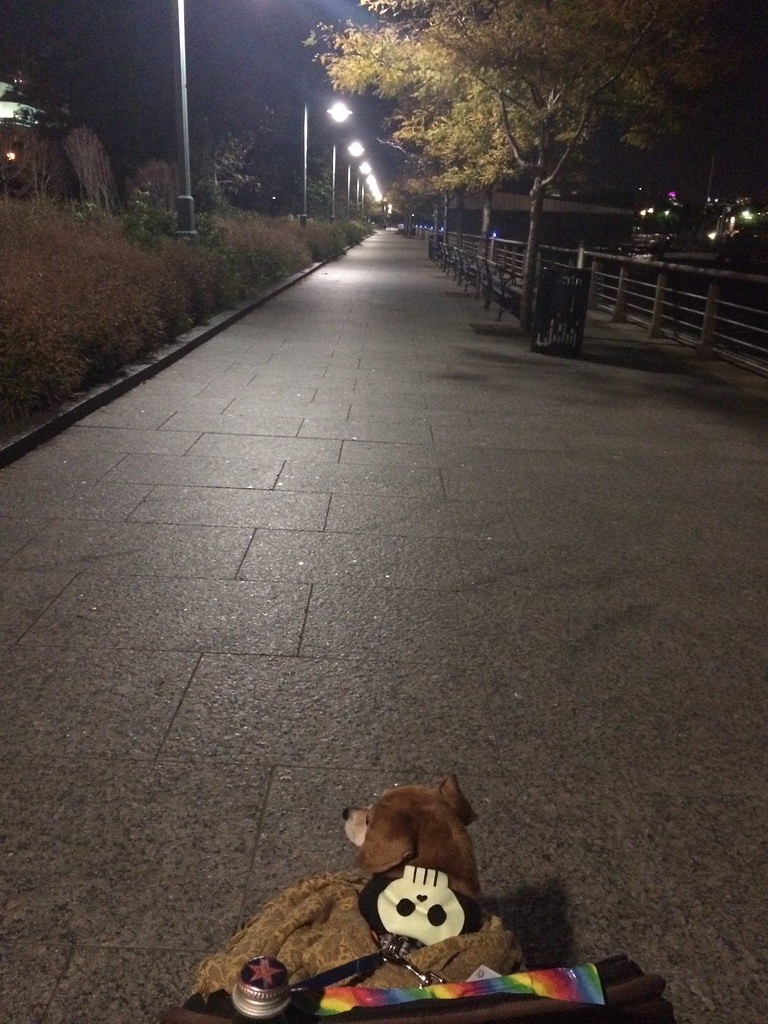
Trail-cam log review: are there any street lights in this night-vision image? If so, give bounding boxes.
[331,137,367,224]
[346,157,384,223]
[301,94,353,228]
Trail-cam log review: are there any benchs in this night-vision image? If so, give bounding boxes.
[430,238,522,321]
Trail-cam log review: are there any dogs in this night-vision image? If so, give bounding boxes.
[338,772,484,950]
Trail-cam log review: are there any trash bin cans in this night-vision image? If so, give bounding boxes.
[412,228,416,236]
[428,234,443,258]
[533,264,591,356]
